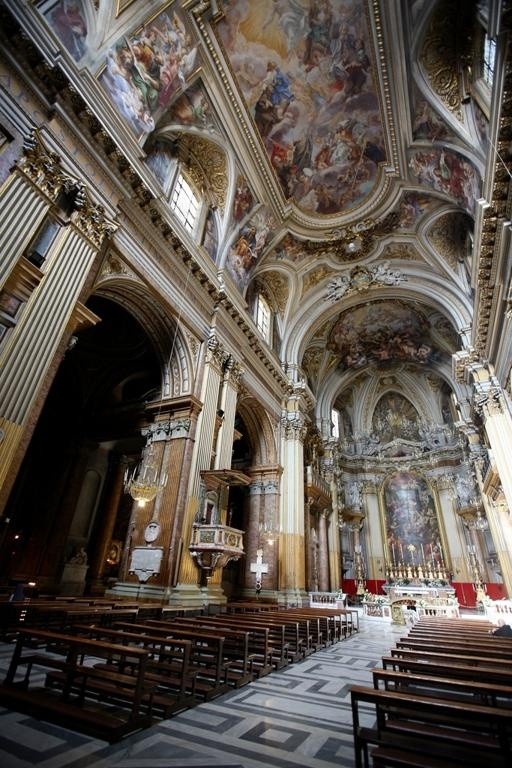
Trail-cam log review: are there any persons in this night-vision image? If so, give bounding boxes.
[339,409,470,564]
[400,600,422,624]
[74,545,88,565]
[488,619,512,636]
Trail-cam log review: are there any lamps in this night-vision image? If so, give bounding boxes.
[122,200,214,507]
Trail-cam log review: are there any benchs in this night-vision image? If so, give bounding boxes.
[350,619,512,768]
[0,597,360,743]
